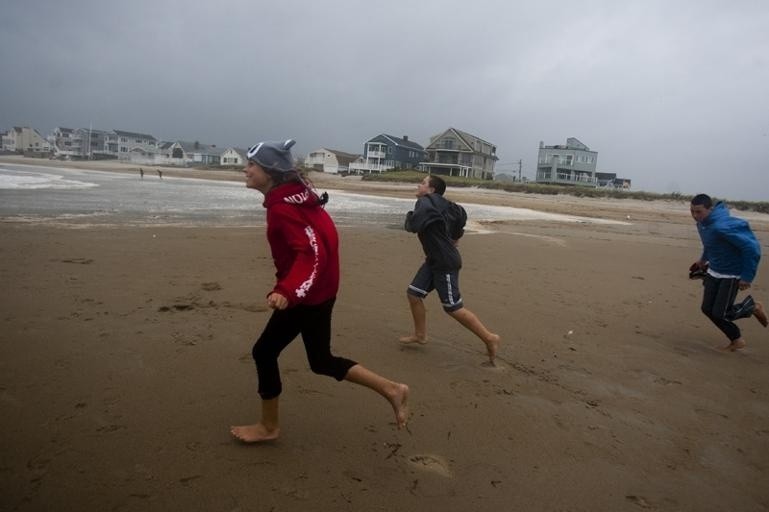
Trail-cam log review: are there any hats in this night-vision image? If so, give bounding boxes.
[245,139,298,174]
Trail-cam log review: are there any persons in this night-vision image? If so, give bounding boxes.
[399,174,500,366]
[226,137,409,443]
[690,194,768,354]
